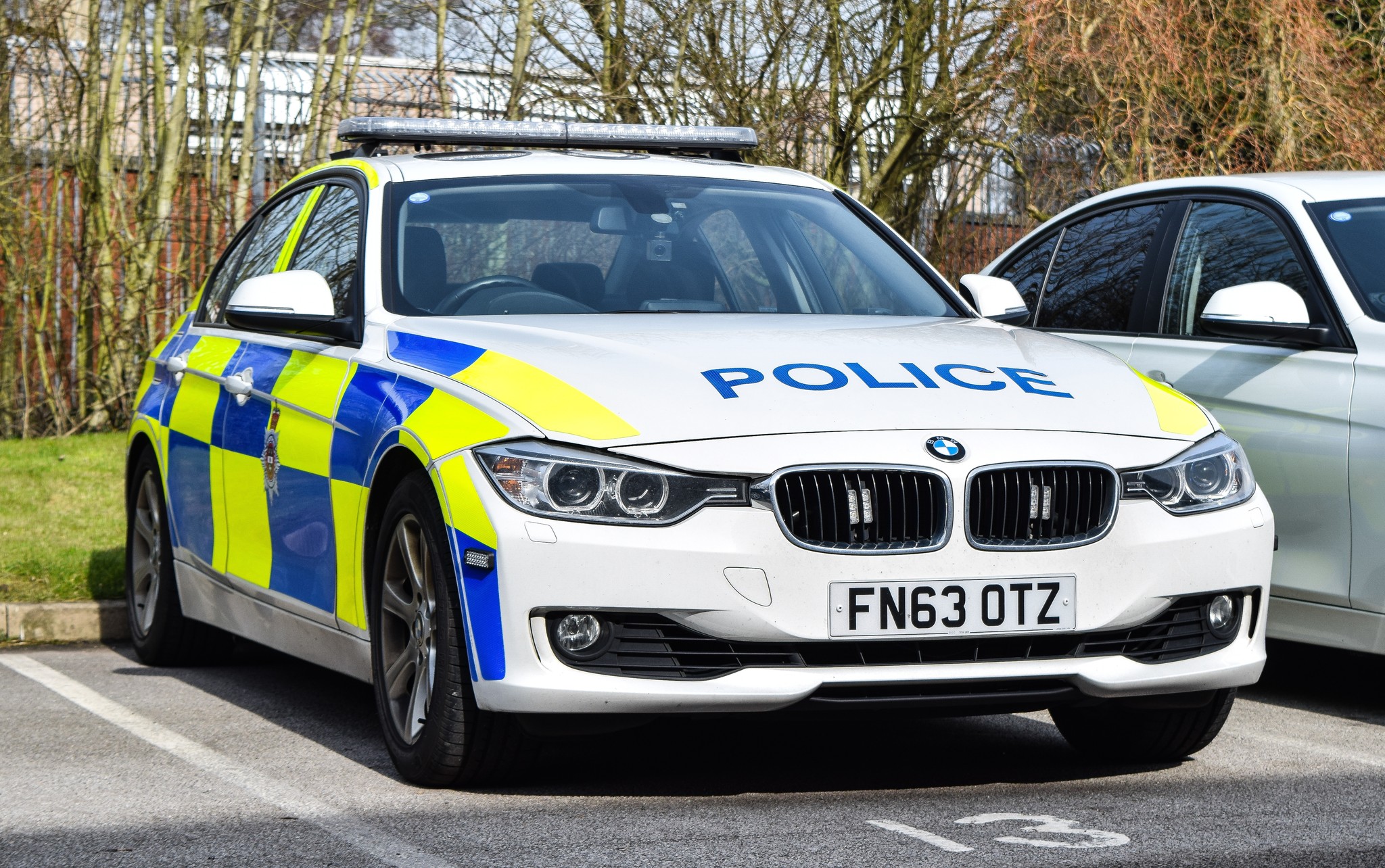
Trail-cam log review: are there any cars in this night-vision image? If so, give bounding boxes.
[119,114,1280,788]
[943,168,1385,665]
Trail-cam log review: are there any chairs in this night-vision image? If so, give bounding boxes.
[404,222,448,313]
[530,260,607,311]
